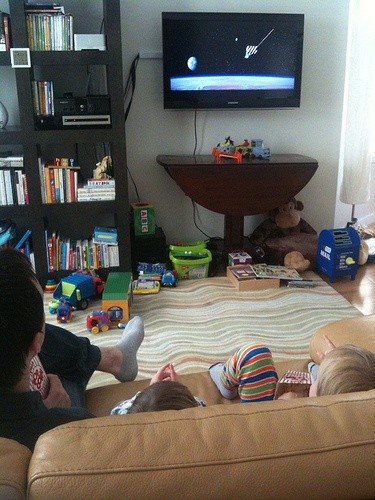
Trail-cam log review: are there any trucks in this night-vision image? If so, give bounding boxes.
[53,273,105,310]
[235,139,272,160]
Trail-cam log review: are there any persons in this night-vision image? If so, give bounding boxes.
[208,334,375,404]
[0,246,146,453]
[110,363,206,417]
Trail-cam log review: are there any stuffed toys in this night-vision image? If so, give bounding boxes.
[249,196,319,273]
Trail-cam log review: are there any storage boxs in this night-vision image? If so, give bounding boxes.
[227,263,281,292]
[169,249,212,280]
[132,202,156,235]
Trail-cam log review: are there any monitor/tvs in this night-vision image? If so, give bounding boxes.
[161,11,304,110]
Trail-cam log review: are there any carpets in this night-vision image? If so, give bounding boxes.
[42,271,363,390]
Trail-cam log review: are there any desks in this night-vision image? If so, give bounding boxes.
[157,154,319,252]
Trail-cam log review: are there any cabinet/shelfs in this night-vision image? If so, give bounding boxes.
[0,0,133,287]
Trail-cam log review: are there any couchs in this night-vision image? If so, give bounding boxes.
[0,314,375,499]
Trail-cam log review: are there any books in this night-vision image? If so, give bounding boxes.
[44,224,121,274]
[0,221,37,274]
[0,11,13,52]
[32,79,55,117]
[37,156,117,204]
[24,1,74,52]
[0,151,30,206]
[230,264,314,289]
